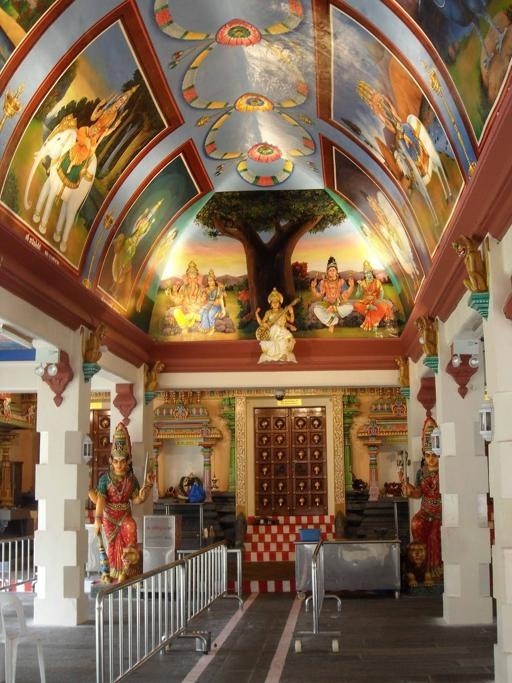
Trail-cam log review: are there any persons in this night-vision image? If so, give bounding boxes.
[253,285,298,363]
[309,255,356,334]
[351,79,421,161]
[43,82,142,175]
[85,421,156,587]
[161,261,206,336]
[354,257,398,336]
[196,269,227,338]
[398,412,444,590]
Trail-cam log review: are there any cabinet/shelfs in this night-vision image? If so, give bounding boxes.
[295,541,400,592]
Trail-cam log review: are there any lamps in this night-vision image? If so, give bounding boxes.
[35,348,61,376]
[431,404,492,458]
[451,339,482,367]
[82,435,93,463]
